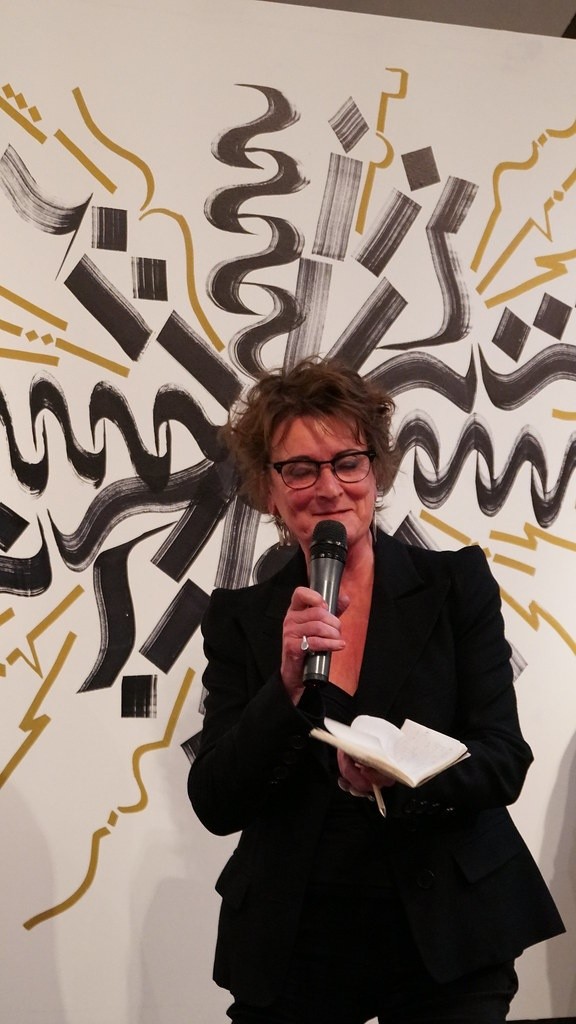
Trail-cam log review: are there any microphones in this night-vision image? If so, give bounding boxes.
[301,519,348,689]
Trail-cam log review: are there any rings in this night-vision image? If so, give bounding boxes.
[367,795,376,802]
[300,635,309,654]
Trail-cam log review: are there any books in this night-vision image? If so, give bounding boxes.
[309,715,473,789]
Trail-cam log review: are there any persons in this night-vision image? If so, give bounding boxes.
[182,354,565,1024]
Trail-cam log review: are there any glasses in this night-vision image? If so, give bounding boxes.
[264,445,376,489]
